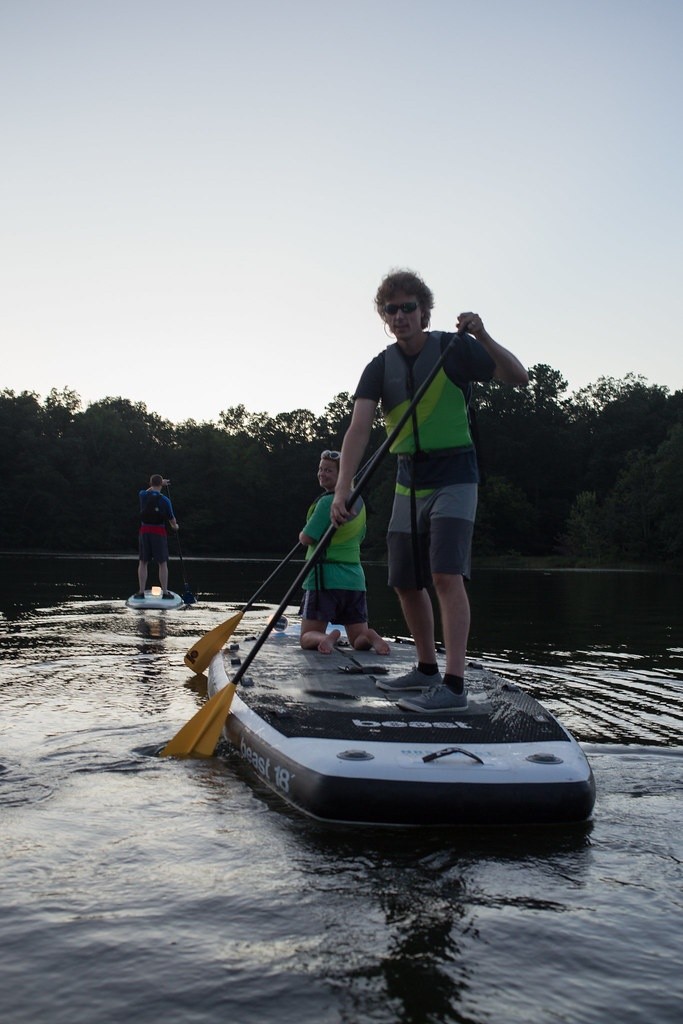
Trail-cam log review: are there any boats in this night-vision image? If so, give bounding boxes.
[125,589,184,610]
[208,621,594,837]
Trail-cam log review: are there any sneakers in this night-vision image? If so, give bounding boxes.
[398,681,468,713]
[375,665,442,690]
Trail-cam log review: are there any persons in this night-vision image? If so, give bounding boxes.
[134,474,179,599]
[330,270,528,714]
[299,452,391,656]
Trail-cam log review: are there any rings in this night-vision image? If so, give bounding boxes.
[471,321,476,327]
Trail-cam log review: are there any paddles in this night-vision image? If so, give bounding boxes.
[181,535,304,678]
[160,318,473,762]
[164,483,196,605]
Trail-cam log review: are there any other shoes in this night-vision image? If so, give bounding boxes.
[162,592,174,599]
[134,592,144,599]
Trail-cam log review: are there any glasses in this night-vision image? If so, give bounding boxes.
[320,450,340,460]
[384,302,421,315]
